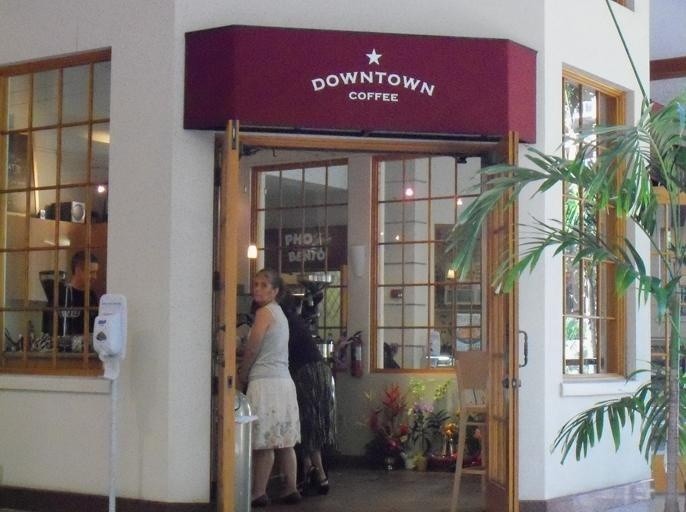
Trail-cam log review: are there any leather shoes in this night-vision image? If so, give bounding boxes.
[249,494,269,508]
[274,492,302,505]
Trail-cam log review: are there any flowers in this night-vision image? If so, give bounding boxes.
[362,377,481,457]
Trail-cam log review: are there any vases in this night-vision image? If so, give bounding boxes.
[383,453,473,471]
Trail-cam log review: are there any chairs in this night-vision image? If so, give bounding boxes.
[451,350,490,510]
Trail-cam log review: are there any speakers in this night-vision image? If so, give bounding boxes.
[50,202,86,224]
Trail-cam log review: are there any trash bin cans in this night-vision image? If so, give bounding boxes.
[234,389,258,512]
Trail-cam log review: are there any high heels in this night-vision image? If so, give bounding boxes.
[311,478,330,497]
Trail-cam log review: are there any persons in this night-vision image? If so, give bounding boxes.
[237,266,302,508]
[249,287,338,495]
[42,250,100,337]
